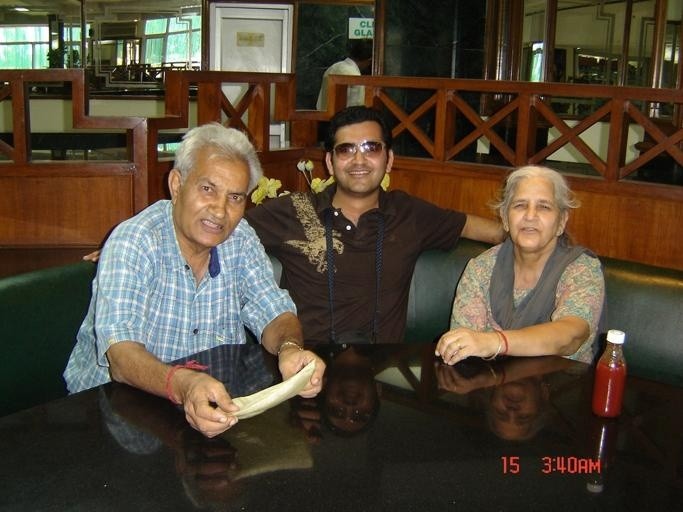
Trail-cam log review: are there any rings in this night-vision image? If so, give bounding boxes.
[451,384,459,391]
[456,341,461,350]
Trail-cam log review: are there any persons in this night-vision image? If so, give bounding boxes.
[277,332,432,437]
[63,119,326,436]
[83,104,514,349]
[426,353,589,445]
[101,380,319,511]
[433,164,607,366]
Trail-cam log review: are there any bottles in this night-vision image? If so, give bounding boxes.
[584,418,618,495]
[591,329,628,416]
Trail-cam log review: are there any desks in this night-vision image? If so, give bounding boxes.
[1,333,682,510]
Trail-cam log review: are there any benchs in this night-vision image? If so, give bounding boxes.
[1,222,683,434]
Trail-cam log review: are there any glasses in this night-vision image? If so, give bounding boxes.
[333,140,386,159]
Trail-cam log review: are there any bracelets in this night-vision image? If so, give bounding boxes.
[275,338,308,353]
[483,329,509,362]
[488,363,505,387]
[166,359,208,405]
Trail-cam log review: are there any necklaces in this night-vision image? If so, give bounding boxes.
[325,212,384,347]
